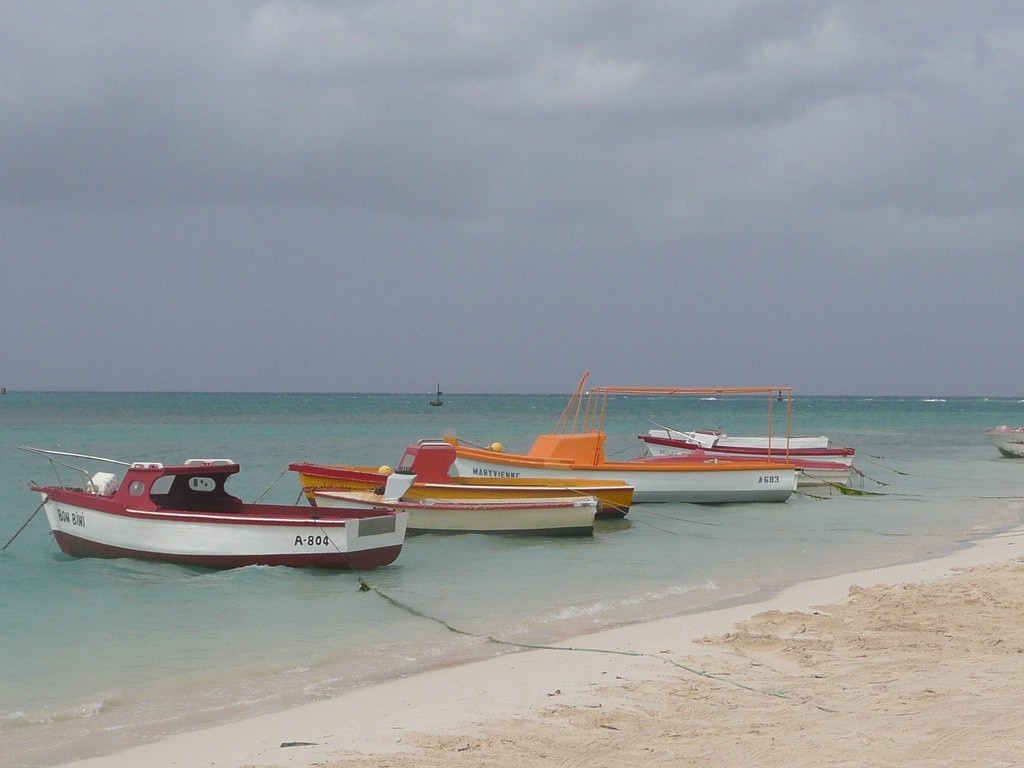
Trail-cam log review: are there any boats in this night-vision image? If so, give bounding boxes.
[982,425,1024,459]
[645,417,831,449]
[15,445,411,573]
[628,447,853,487]
[442,369,805,504]
[310,471,601,538]
[285,438,637,520]
[638,433,856,469]
